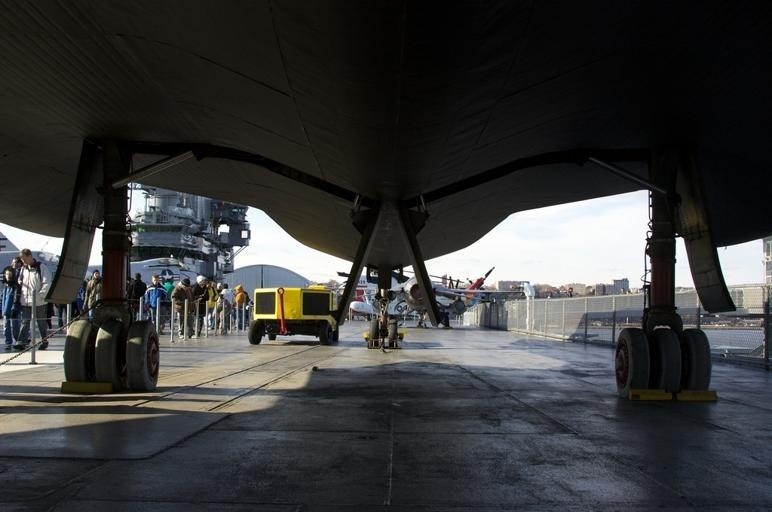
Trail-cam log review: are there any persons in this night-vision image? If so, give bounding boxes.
[2,248,102,351]
[127,273,253,339]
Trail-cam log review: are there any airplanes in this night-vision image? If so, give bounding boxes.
[0,0,772,397]
[338,274,524,327]
[129,258,200,288]
[0,231,95,283]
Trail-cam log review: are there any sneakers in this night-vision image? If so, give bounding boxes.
[39,342,48,349]
[6,340,31,350]
[179,335,196,339]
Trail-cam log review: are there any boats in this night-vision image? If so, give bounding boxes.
[130,183,251,285]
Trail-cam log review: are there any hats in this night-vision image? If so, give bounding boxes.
[181,279,190,287]
[197,275,206,284]
